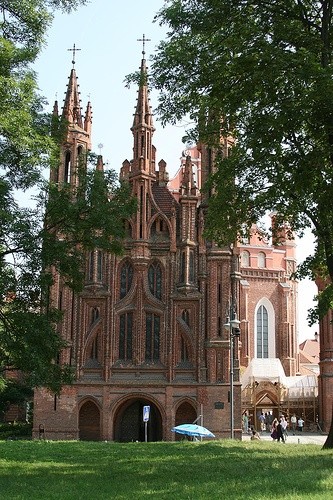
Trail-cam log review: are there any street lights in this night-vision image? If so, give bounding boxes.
[224,307,241,439]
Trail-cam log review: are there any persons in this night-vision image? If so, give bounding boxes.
[243,412,251,436]
[298,418,305,433]
[276,420,288,443]
[259,413,265,431]
[267,411,272,432]
[270,420,279,443]
[264,413,268,431]
[279,415,287,441]
[251,430,260,440]
[291,414,297,436]
[272,417,279,424]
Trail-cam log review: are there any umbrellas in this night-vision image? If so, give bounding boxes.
[171,423,214,438]
